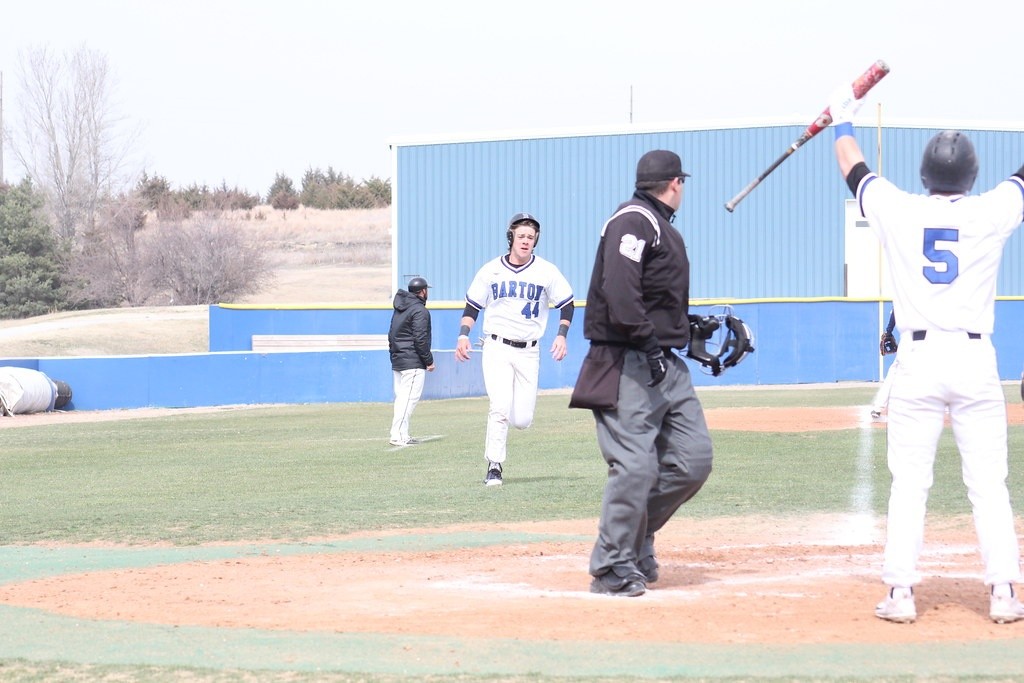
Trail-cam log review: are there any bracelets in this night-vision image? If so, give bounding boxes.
[458,335,469,341]
[835,123,854,140]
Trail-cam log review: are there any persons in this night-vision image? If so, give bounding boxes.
[568,150,712,598]
[871,358,897,418]
[830,91,1024,625]
[388,277,436,446]
[456,212,574,486]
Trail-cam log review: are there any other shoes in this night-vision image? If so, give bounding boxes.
[871,410,880,417]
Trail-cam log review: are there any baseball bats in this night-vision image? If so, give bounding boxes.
[723,57,892,213]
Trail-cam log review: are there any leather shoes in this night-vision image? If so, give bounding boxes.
[645,567,658,581]
[590,576,644,596]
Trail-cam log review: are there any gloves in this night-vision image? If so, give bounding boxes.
[647,347,667,386]
[829,83,863,125]
[689,313,719,338]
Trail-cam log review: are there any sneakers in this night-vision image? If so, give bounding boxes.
[485,463,502,484]
[875,596,916,623]
[988,594,1024,623]
[390,437,420,445]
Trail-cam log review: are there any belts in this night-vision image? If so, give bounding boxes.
[492,335,537,347]
[913,330,981,340]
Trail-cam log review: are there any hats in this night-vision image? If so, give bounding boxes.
[636,150,691,180]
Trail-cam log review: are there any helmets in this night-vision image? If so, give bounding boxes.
[507,212,540,246]
[920,131,977,191]
[408,277,432,292]
